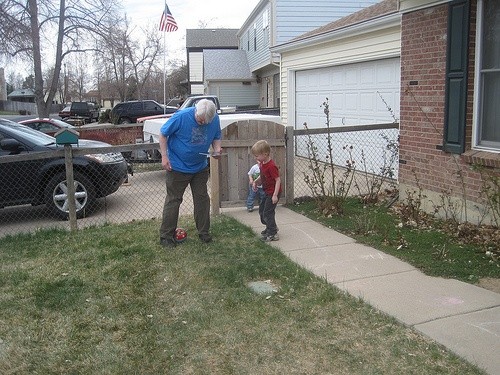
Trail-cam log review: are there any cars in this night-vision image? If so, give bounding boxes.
[58,101,101,123]
[16,117,76,134]
[0,119,128,222]
[178,96,220,111]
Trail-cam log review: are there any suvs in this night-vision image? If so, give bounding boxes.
[109,100,178,124]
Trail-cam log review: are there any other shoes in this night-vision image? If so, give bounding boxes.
[159,237,176,247]
[247,208,253,212]
[198,234,212,243]
[261,229,279,241]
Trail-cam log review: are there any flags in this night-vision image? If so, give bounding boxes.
[159,3,178,32]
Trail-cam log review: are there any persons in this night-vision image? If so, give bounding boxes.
[158,99,222,247]
[247,160,265,212]
[249,140,282,241]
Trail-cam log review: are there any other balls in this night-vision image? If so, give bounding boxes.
[175,228,187,242]
